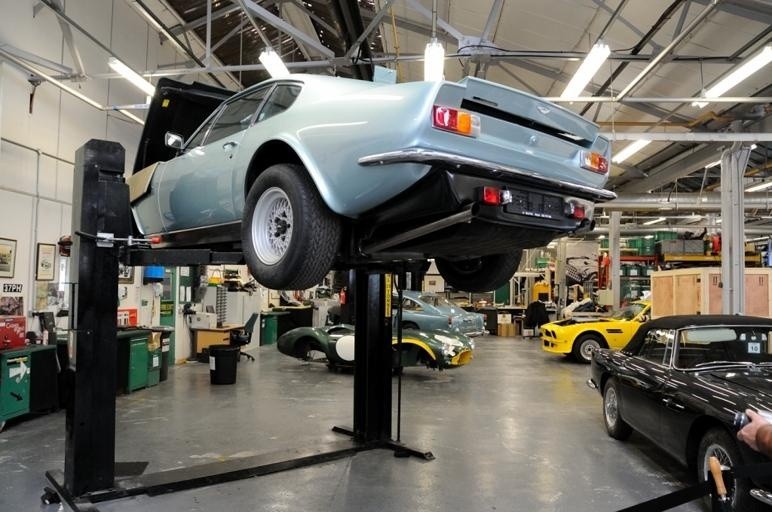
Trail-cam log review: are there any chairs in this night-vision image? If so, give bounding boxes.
[522,301,548,340]
[231,312,259,361]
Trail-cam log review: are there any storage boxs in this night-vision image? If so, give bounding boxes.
[619,265,656,276]
[656,239,705,258]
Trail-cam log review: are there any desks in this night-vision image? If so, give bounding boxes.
[189,324,246,360]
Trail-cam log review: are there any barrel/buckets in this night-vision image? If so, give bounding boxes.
[208,345,239,385]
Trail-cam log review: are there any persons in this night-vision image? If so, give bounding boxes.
[736,409,772,458]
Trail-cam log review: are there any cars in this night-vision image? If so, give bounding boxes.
[324,290,491,338]
[567,252,600,289]
[590,313,771,511]
[540,301,652,365]
[125,74,616,294]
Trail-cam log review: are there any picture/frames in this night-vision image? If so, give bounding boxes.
[35,243,56,280]
[0,238,17,278]
[118,262,135,284]
